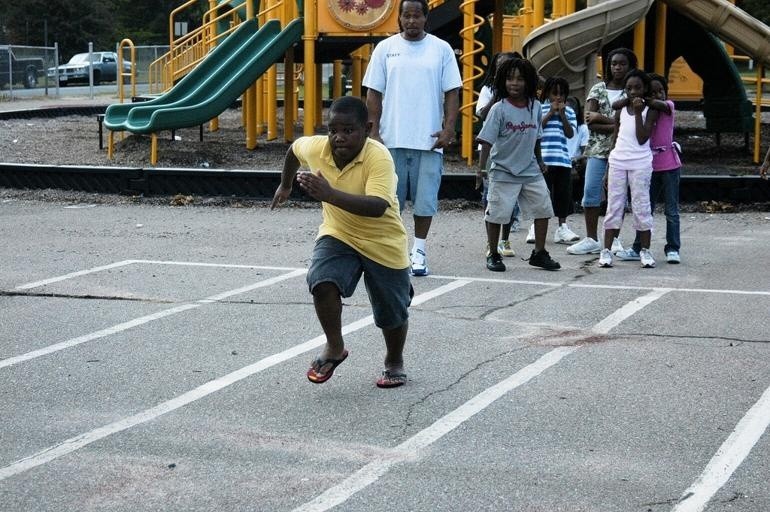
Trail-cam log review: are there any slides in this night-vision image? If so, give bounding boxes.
[102,17,303,134]
[520,0,770,134]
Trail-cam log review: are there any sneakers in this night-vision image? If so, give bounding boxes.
[486,251,506,272]
[639,247,656,269]
[528,249,561,270]
[565,236,602,255]
[598,248,614,268]
[665,249,680,264]
[615,247,642,261]
[497,237,516,257]
[610,235,624,255]
[409,248,429,276]
[526,223,536,245]
[553,222,581,243]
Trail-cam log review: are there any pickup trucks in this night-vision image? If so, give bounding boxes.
[0,45,46,89]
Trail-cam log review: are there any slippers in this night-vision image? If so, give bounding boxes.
[307,348,349,384]
[375,367,407,388]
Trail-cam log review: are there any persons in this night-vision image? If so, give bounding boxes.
[474,50,526,258]
[268,93,416,392]
[595,66,660,270]
[564,46,640,259]
[610,71,686,265]
[472,57,563,273]
[563,95,592,220]
[360,0,465,280]
[525,75,582,245]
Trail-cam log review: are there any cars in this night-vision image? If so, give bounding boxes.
[45,49,138,87]
